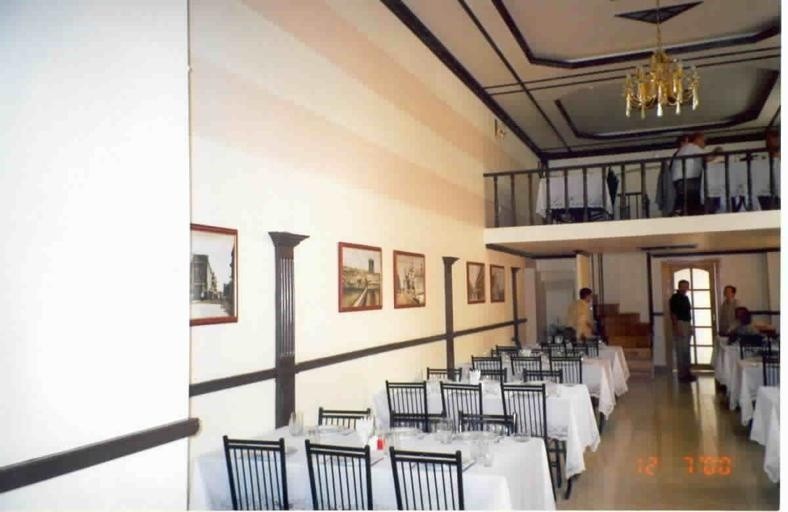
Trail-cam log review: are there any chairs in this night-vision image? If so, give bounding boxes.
[656,158,690,216]
[198,333,630,510]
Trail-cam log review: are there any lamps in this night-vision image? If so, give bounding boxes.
[623,0,700,120]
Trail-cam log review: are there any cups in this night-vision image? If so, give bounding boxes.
[285,408,504,471]
[416,335,602,398]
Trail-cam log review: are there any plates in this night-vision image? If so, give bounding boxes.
[510,432,531,443]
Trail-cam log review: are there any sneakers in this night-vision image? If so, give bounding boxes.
[678,373,698,384]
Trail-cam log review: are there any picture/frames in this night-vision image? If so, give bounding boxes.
[189,223,239,326]
[338,241,383,313]
[490,264,505,302]
[393,249,426,309]
[466,261,486,304]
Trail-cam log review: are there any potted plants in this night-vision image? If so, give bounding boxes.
[548,315,567,344]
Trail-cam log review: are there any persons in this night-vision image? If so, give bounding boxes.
[669,134,689,166]
[765,131,780,158]
[728,307,768,347]
[568,288,596,340]
[719,286,742,336]
[670,280,697,382]
[670,133,723,216]
